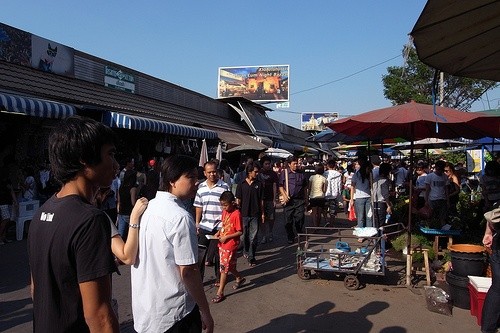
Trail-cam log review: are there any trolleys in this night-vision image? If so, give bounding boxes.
[295,222,406,290]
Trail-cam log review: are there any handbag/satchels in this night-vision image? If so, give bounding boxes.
[418,206,433,218]
[348,206,357,223]
[279,191,290,207]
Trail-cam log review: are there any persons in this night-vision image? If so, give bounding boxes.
[193,155,277,303]
[94,154,172,260]
[27,113,121,333]
[273,146,460,260]
[131,154,215,333]
[89,184,148,265]
[0,157,64,246]
[478,150,500,333]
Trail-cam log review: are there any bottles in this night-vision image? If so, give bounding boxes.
[384,213,391,224]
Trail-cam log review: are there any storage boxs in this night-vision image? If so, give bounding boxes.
[420,222,463,234]
[466,275,493,326]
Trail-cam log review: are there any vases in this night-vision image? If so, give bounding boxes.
[22,187,34,200]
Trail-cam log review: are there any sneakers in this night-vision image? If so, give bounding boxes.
[323,221,330,227]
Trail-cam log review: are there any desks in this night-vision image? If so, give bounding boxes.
[13,199,40,217]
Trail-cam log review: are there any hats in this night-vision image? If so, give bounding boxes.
[371,155,382,165]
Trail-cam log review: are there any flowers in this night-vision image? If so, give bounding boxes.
[16,176,38,188]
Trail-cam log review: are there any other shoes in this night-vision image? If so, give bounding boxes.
[260,240,266,243]
[268,233,273,242]
[289,241,293,244]
[0,240,6,245]
[6,239,13,242]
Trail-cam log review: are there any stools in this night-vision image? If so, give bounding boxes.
[407,247,430,286]
[435,236,453,261]
[15,216,35,241]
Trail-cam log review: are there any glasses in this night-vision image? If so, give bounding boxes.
[444,170,450,173]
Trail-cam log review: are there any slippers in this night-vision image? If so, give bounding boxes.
[214,280,220,287]
[248,263,257,268]
[212,293,224,302]
[233,278,246,289]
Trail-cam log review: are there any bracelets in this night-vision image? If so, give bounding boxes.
[129,223,139,228]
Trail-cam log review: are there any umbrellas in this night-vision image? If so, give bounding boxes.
[408,0,500,82]
[373,138,500,160]
[304,129,372,143]
[199,139,208,167]
[223,143,264,153]
[216,142,222,169]
[324,100,500,286]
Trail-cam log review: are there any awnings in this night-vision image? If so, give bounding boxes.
[0,92,76,119]
[101,111,218,140]
[251,135,319,154]
[200,126,268,151]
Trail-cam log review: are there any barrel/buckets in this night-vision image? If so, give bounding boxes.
[446,243,487,277]
[445,271,471,309]
